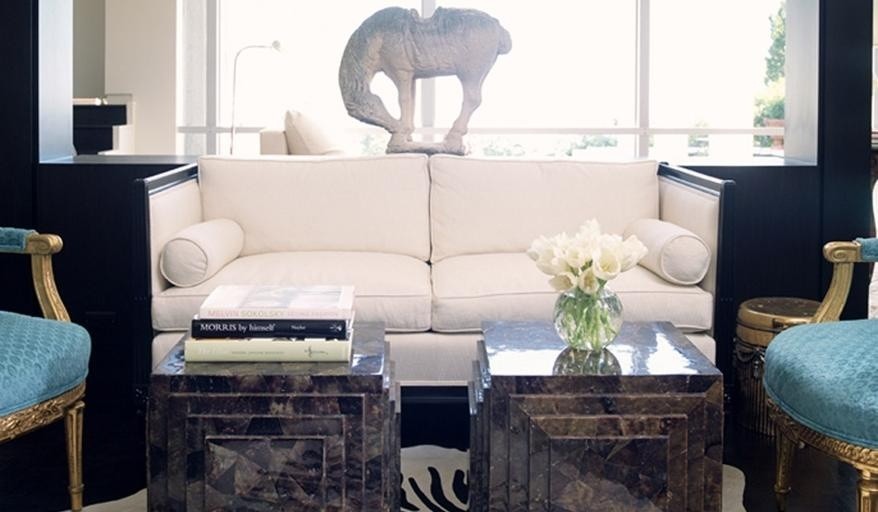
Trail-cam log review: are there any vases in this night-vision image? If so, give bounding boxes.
[551,287,624,352]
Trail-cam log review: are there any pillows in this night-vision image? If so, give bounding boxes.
[196,153,432,262]
[624,216,712,286]
[430,154,662,267]
[285,107,334,154]
[158,217,245,289]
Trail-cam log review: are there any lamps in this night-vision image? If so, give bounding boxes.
[228,26,297,154]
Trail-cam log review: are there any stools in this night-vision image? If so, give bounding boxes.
[732,296,842,438]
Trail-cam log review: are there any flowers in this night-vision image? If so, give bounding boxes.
[526,218,649,294]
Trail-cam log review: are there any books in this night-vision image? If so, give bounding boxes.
[199,283,352,318]
[190,311,356,340]
[184,329,354,362]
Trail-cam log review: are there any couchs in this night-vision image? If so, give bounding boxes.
[132,160,737,512]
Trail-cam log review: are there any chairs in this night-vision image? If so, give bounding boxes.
[762,236,878,511]
[0,228,93,511]
